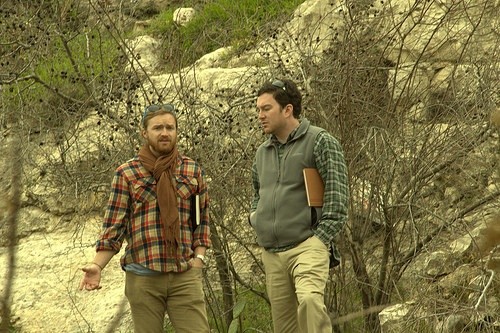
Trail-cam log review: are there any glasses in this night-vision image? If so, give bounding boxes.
[145,104,175,117]
[272,79,291,98]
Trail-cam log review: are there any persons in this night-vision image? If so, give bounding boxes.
[249,77,348,332]
[80,104,213,333]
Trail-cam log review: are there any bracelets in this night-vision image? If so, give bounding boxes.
[196,254,207,265]
[94,263,102,271]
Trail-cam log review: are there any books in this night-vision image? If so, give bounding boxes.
[302,168,325,207]
